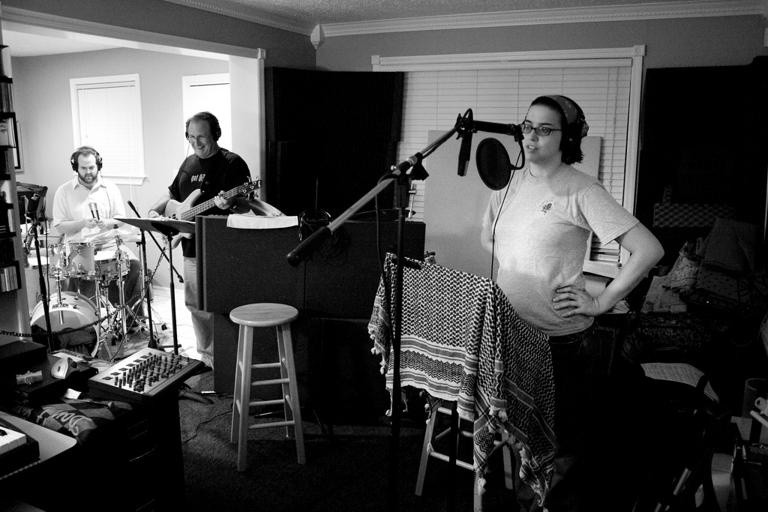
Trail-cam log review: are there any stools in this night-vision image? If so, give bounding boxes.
[229,303,307,471]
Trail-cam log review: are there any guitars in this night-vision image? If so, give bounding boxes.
[163,175,262,250]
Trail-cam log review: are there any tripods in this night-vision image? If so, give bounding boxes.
[49,249,213,408]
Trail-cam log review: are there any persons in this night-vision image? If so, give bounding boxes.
[480,93,664,512]
[147,110,254,368]
[52,145,149,338]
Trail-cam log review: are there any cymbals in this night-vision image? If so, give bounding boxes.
[91,224,140,244]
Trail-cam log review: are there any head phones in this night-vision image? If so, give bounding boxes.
[185,112,221,141]
[70,147,104,172]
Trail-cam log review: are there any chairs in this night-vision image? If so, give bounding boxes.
[385,251,552,511]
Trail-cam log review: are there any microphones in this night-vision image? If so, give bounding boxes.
[456,108,477,176]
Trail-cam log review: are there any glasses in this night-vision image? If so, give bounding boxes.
[520,124,561,136]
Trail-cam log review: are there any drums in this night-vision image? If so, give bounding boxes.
[67,237,96,281]
[30,292,101,361]
[94,247,129,278]
